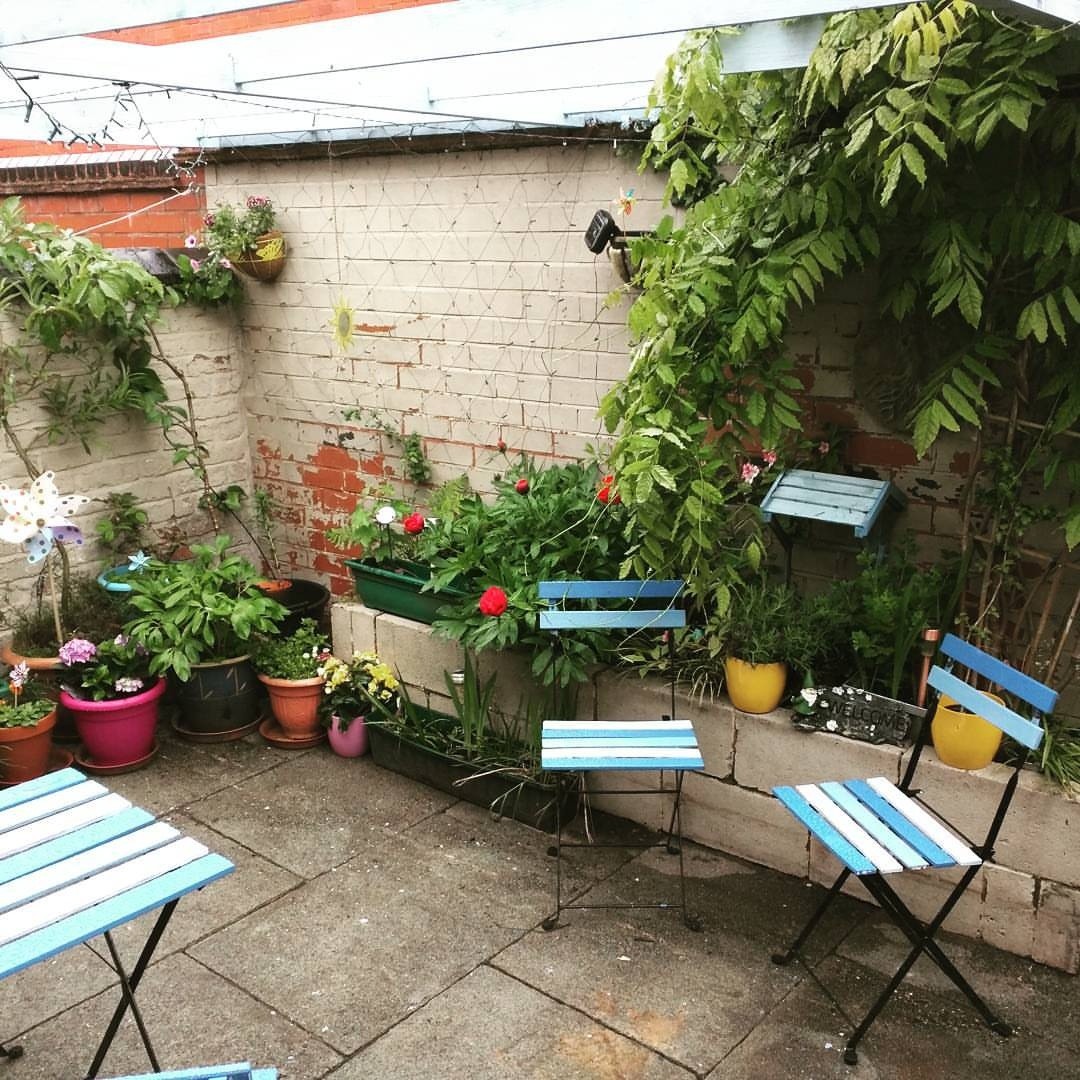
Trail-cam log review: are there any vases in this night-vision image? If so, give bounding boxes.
[1,708,56,784]
[256,577,331,642]
[230,233,285,283]
[2,618,124,699]
[59,671,165,777]
[256,672,330,750]
[327,713,371,760]
[345,557,475,627]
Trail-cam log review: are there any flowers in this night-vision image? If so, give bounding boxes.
[317,648,400,731]
[1,196,234,729]
[261,619,334,682]
[329,409,648,690]
[180,198,278,275]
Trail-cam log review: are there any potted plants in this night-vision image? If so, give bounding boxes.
[930,447,1057,772]
[365,648,596,834]
[128,536,286,745]
[714,577,830,714]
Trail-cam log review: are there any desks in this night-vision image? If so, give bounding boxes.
[0,767,235,1079]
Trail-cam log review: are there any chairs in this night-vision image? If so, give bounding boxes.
[770,624,1063,1066]
[539,581,702,930]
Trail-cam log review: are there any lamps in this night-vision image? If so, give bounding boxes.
[583,209,655,255]
